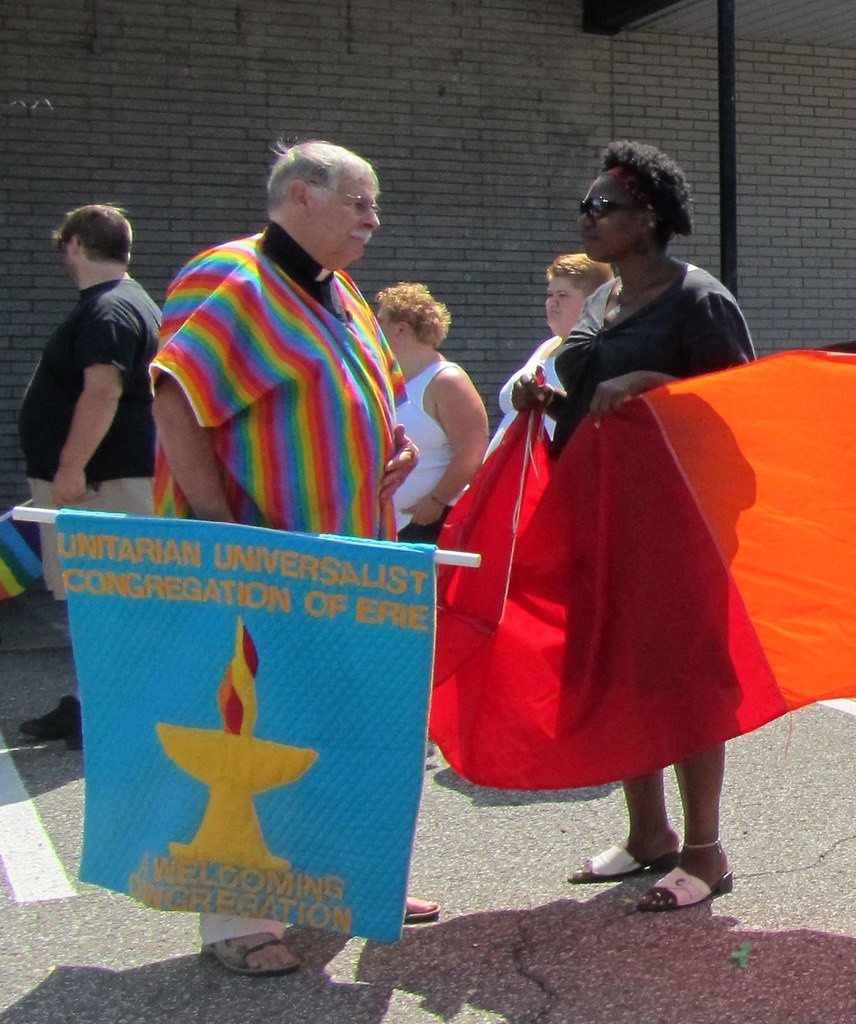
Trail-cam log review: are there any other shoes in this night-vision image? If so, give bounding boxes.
[426,753,441,769]
[65,734,83,750]
[19,695,82,738]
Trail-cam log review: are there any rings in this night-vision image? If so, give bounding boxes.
[401,448,415,460]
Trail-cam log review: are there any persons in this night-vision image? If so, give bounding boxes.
[483,254,613,467]
[511,140,757,911]
[19,205,160,750]
[149,142,440,974]
[375,286,489,546]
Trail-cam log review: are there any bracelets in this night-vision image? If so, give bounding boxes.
[431,495,447,506]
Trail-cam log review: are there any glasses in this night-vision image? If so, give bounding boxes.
[580,196,655,219]
[304,179,378,214]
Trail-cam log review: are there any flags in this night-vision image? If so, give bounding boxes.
[0,521,44,598]
[54,513,437,943]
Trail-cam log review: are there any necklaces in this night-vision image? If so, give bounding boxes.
[607,255,671,322]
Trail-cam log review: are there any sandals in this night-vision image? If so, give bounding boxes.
[201,933,302,976]
[406,897,440,920]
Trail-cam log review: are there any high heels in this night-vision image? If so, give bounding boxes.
[567,846,679,884]
[638,866,733,909]
